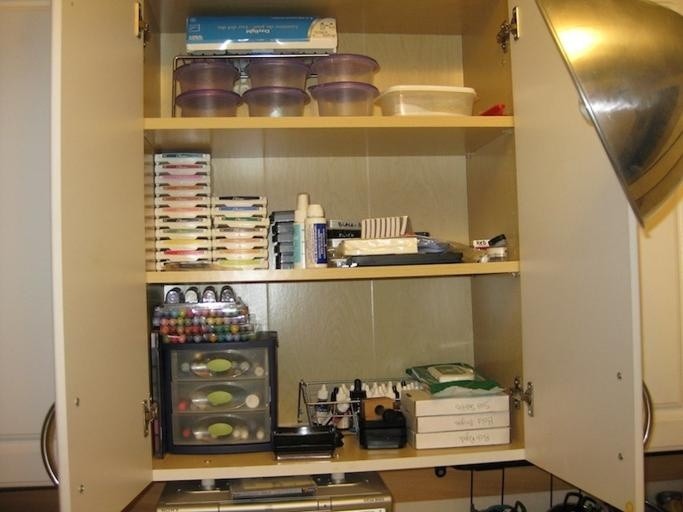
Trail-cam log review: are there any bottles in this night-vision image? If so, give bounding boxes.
[314,379,400,434]
[293,194,328,270]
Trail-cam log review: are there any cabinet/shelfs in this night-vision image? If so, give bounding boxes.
[50,0,645,512]
